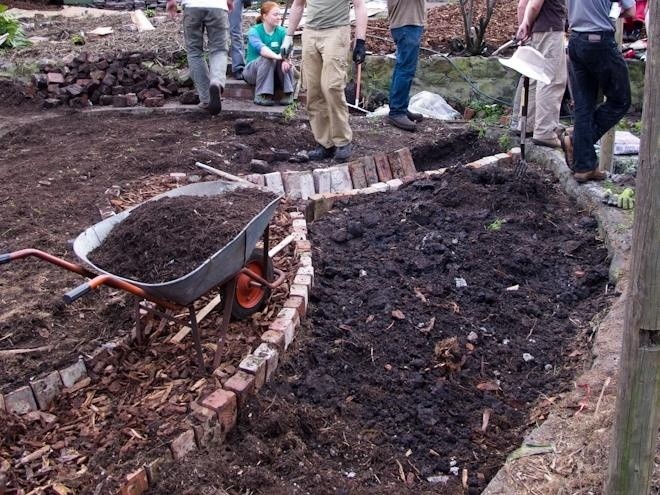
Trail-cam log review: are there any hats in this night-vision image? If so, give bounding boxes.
[496,44,552,86]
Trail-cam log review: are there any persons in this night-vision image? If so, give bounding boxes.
[242,1,296,105]
[228,0,252,80]
[280,0,368,161]
[386,0,427,130]
[499,0,650,183]
[166,0,234,115]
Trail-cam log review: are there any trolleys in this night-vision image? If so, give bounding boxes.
[0,175,290,385]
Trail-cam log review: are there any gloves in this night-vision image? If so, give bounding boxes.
[352,37,366,66]
[280,34,294,59]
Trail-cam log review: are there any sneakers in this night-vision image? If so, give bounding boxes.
[253,94,276,106]
[279,91,294,106]
[207,82,222,115]
[561,134,609,184]
[407,110,425,122]
[335,140,354,161]
[308,144,334,160]
[389,112,417,132]
